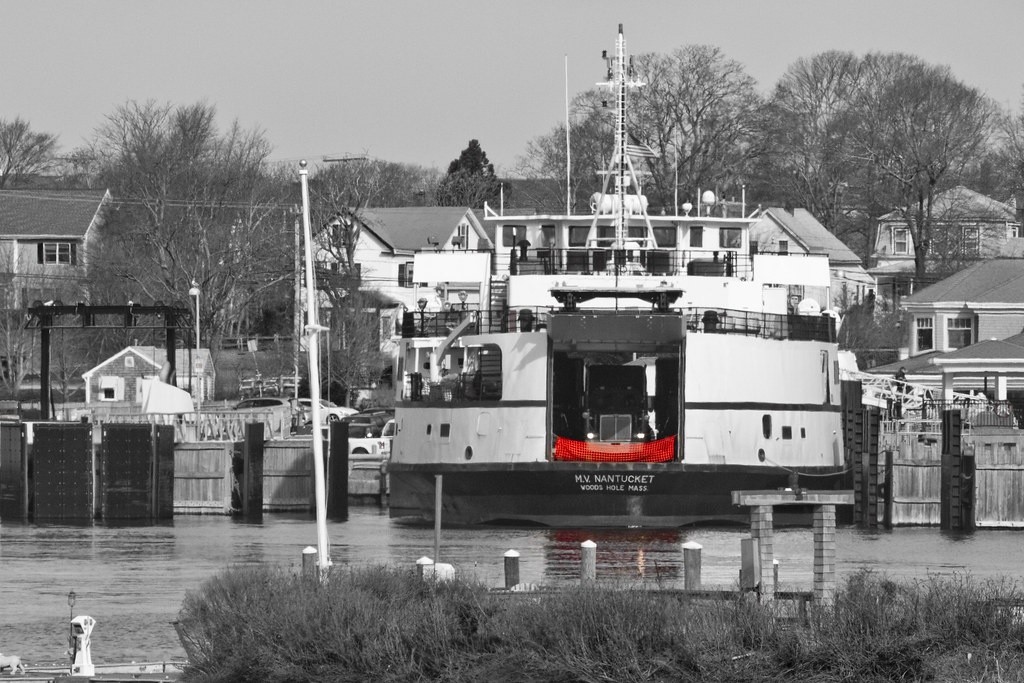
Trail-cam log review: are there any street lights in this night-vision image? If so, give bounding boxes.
[458,289,468,311]
[188,288,201,412]
[417,297,428,334]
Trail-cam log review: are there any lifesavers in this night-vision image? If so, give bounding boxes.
[994,400,1013,418]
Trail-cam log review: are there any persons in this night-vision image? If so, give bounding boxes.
[893,366,910,420]
[864,288,876,315]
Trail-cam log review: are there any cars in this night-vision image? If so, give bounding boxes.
[334,406,397,460]
[298,398,359,422]
[231,398,306,429]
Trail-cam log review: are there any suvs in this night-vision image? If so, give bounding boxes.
[0,399,25,422]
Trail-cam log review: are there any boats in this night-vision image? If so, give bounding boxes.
[381,24,861,541]
